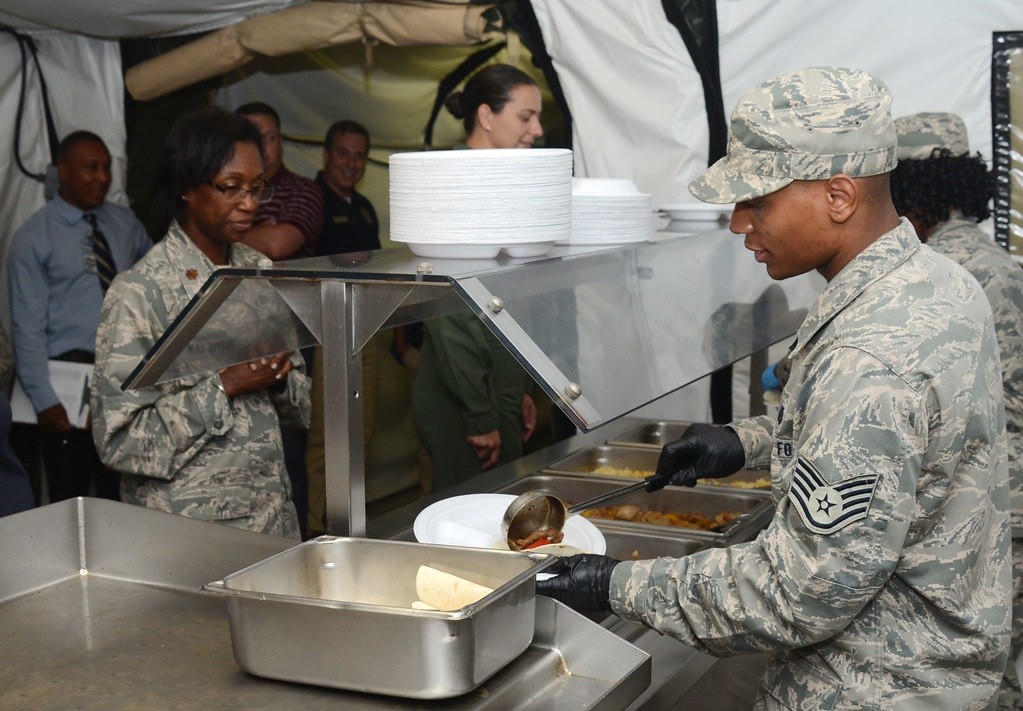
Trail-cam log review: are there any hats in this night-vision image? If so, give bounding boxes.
[891,112,971,162]
[689,65,898,203]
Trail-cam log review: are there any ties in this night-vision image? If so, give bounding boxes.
[83,213,117,298]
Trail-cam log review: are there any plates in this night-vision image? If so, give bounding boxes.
[388,148,737,260]
[413,493,607,583]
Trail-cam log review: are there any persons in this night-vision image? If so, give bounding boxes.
[85,103,317,546]
[532,65,1023,710]
[236,95,382,540]
[6,131,156,503]
[888,111,1022,709]
[390,63,551,490]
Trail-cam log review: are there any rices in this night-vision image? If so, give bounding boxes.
[592,466,774,488]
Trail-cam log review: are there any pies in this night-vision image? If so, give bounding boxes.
[519,543,587,557]
[411,565,496,610]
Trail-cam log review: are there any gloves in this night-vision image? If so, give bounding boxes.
[536,553,622,620]
[646,423,743,493]
[761,363,781,389]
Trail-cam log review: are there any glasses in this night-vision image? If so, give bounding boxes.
[213,180,275,205]
